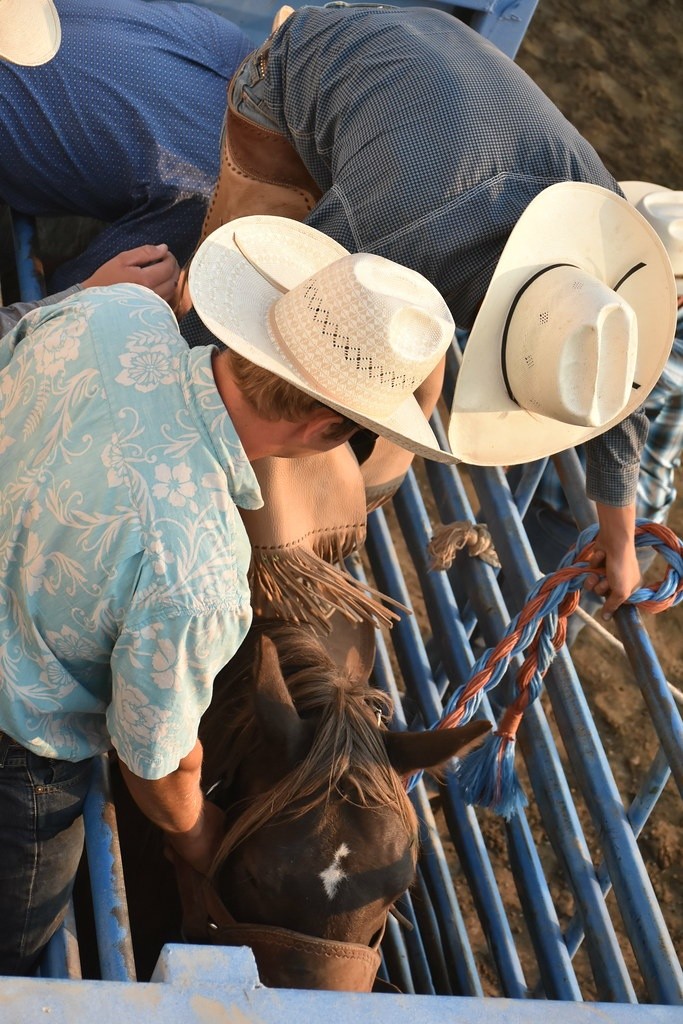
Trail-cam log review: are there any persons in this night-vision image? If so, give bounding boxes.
[0,0,683,623]
[0,216,460,975]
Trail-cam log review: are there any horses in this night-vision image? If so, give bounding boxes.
[156,439,493,992]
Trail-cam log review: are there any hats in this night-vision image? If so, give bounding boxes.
[0,0,62,66]
[188,216,462,468]
[618,180,683,296]
[448,182,677,466]
